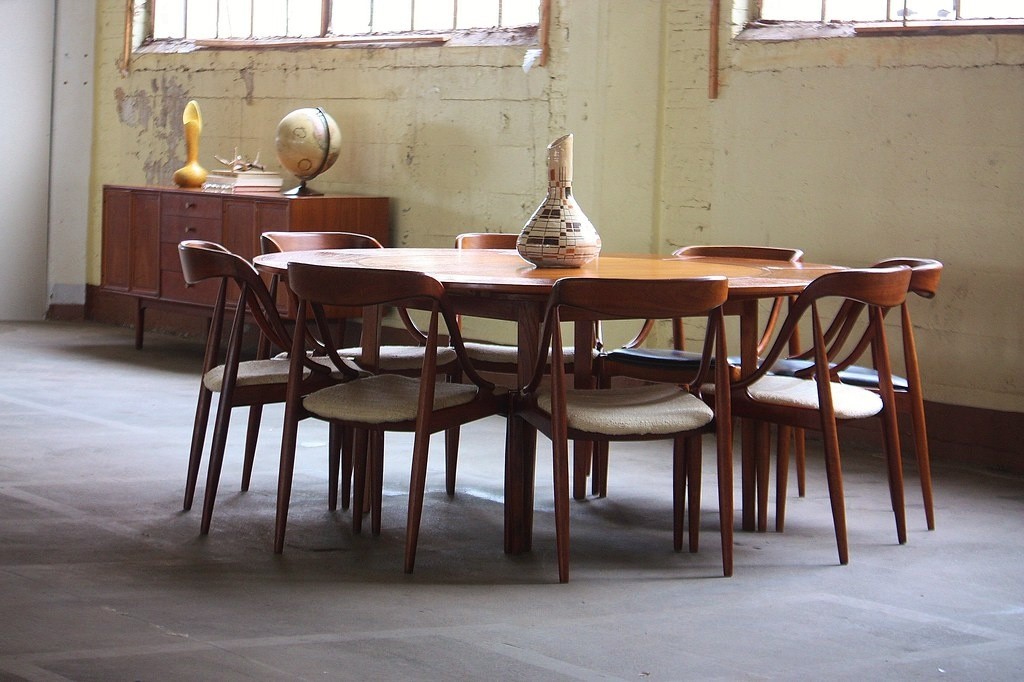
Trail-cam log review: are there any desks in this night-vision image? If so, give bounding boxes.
[252,245,851,558]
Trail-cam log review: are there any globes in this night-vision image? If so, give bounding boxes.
[274,106,341,196]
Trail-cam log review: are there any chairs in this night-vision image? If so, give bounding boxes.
[273,259,512,576]
[766,256,942,532]
[261,227,459,508]
[445,230,602,499]
[598,245,806,498]
[177,237,380,541]
[685,265,911,568]
[503,275,736,583]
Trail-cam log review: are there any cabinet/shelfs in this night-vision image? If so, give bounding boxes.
[98,184,392,356]
